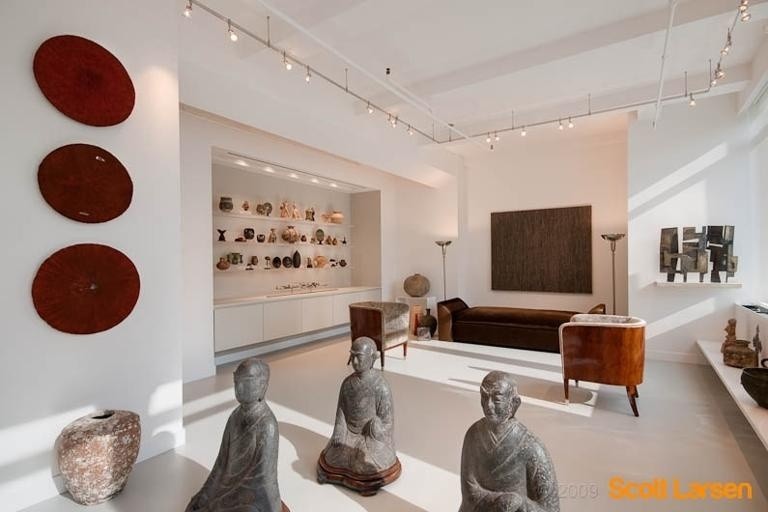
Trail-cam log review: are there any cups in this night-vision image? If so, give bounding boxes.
[257,234,265,242]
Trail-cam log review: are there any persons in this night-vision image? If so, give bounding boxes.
[461,371,558,511]
[185,358,283,512]
[324,336,397,477]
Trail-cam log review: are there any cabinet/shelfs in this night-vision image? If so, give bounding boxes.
[212,196,356,274]
[215,285,382,359]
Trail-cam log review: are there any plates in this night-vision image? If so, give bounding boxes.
[272,256,292,268]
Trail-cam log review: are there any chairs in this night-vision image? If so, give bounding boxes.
[346,300,412,370]
[556,311,647,417]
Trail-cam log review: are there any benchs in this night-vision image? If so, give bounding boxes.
[436,295,606,362]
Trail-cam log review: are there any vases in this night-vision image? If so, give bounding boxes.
[419,308,438,338]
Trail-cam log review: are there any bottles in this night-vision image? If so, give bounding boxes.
[265,256,271,269]
